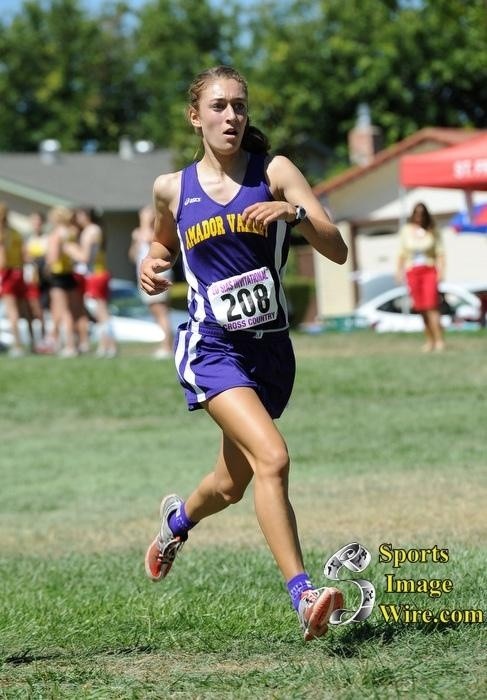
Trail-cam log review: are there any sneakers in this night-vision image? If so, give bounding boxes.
[145,494,188,583]
[299,587,344,641]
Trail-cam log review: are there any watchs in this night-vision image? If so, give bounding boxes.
[284,204,308,229]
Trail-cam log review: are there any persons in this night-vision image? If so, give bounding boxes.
[138,64,351,643]
[128,203,176,361]
[0,199,122,361]
[391,201,449,353]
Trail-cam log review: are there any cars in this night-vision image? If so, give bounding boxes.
[357,283,484,332]
[0,274,188,357]
